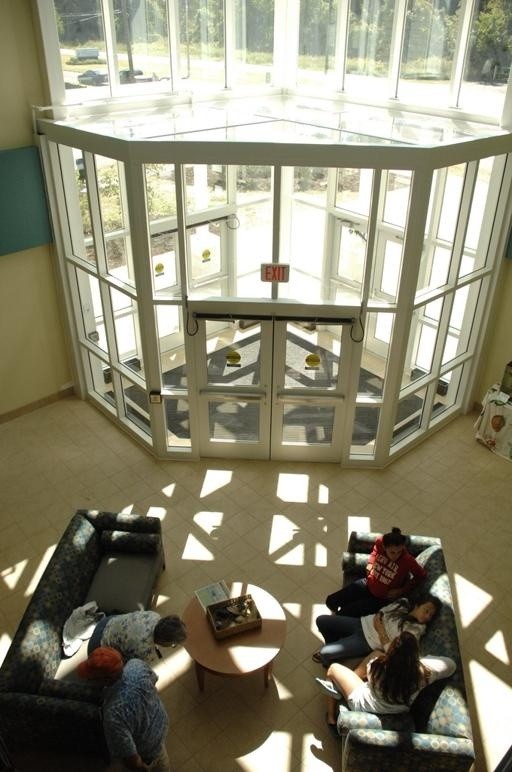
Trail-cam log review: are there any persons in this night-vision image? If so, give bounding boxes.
[315,631,457,736]
[312,593,443,670]
[78,647,170,772]
[326,528,426,617]
[87,610,187,665]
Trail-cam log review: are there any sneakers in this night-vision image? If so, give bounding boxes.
[312,651,324,663]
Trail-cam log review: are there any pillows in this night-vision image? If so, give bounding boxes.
[98,529,164,560]
[336,704,418,739]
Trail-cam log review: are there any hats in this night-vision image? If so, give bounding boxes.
[76,647,124,679]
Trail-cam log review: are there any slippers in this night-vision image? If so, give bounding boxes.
[316,678,344,702]
[325,712,339,739]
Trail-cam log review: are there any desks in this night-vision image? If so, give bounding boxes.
[474,382,512,463]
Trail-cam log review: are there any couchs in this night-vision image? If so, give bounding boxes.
[337,527,476,772]
[2,506,168,771]
